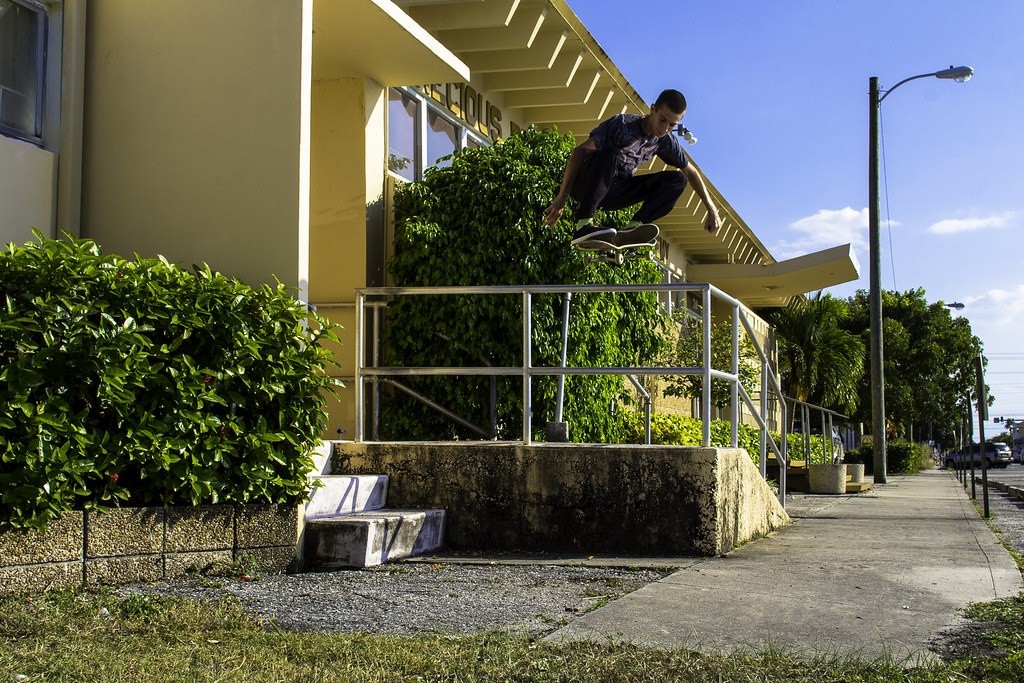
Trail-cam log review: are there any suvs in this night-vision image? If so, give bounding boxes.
[944,440,1015,469]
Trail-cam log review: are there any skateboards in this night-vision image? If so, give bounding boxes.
[575,237,657,266]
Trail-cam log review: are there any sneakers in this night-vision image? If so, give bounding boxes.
[570,223,617,246]
[613,222,660,247]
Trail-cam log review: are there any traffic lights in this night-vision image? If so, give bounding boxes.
[1001,417,1003,422]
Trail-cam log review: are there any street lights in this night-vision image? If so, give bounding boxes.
[864,64,974,481]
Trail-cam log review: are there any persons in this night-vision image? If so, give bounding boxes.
[543,89,722,247]
